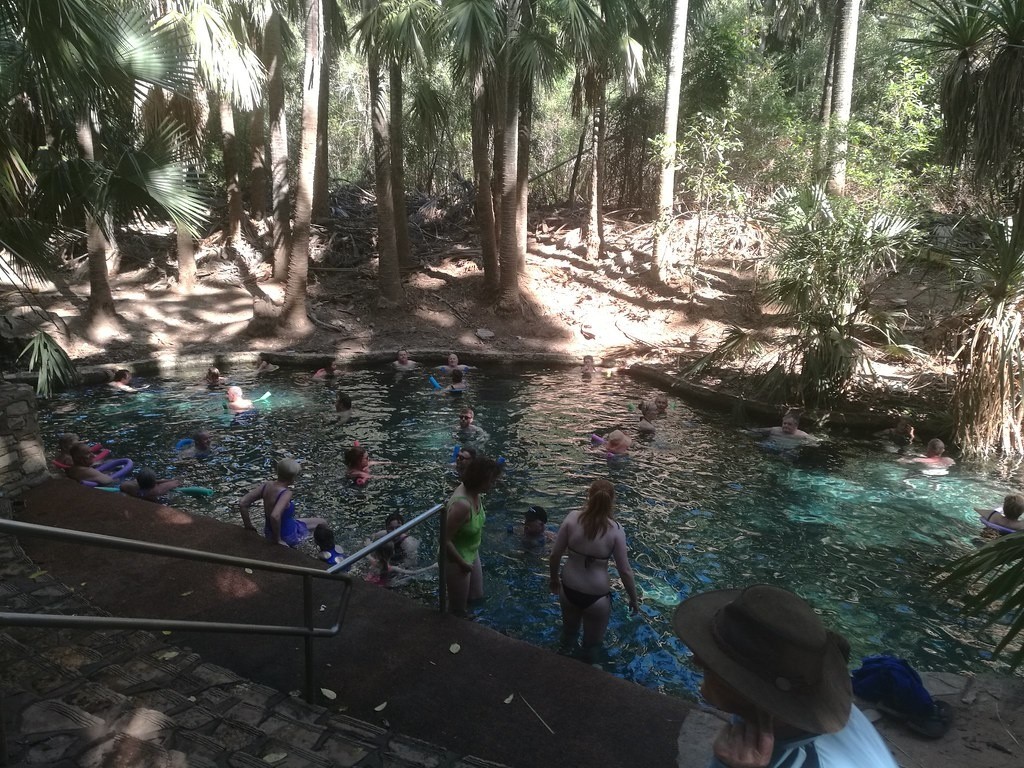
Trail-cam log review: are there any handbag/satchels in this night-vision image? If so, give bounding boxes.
[851,653,935,725]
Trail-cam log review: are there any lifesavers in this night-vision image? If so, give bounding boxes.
[978,516,1017,534]
[627,400,676,418]
[429,376,465,393]
[506,524,559,545]
[93,486,214,498]
[591,433,614,458]
[222,390,272,416]
[80,457,134,487]
[452,442,506,466]
[51,443,111,469]
[175,438,212,458]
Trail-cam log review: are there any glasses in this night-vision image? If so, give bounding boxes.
[455,454,471,461]
[362,456,370,461]
[459,415,471,420]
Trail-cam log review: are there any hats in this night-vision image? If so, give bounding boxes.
[672,584,852,734]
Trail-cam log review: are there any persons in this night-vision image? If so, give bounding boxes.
[448,370,467,389]
[882,416,952,463]
[239,458,324,546]
[224,386,250,408]
[313,524,343,562]
[451,448,476,466]
[393,349,417,366]
[607,396,671,454]
[108,369,131,387]
[204,367,224,382]
[254,352,273,370]
[363,513,414,583]
[334,394,356,410]
[769,413,809,437]
[672,584,900,768]
[317,356,342,376]
[576,355,600,371]
[443,353,467,369]
[438,457,641,647]
[457,407,478,427]
[67,445,113,484]
[977,493,1024,530]
[195,430,211,450]
[57,432,90,464]
[121,468,178,497]
[344,448,372,475]
